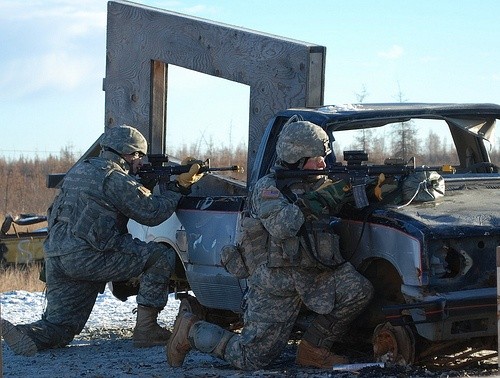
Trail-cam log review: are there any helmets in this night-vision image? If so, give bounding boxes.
[276,121,332,164]
[100,126,147,155]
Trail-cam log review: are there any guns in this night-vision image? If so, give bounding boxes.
[132,154,240,195]
[275,150,441,210]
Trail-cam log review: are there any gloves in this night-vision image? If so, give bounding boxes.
[303,176,354,214]
[138,163,157,191]
[176,156,205,189]
[370,157,404,200]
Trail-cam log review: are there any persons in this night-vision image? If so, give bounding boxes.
[0,124,207,356]
[166,120,400,372]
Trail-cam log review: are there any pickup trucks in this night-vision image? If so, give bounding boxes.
[108,103,500,365]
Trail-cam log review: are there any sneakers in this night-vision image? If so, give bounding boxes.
[295,338,350,370]
[167,309,203,367]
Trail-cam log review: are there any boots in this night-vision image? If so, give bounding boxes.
[0,318,42,356]
[132,304,173,344]
